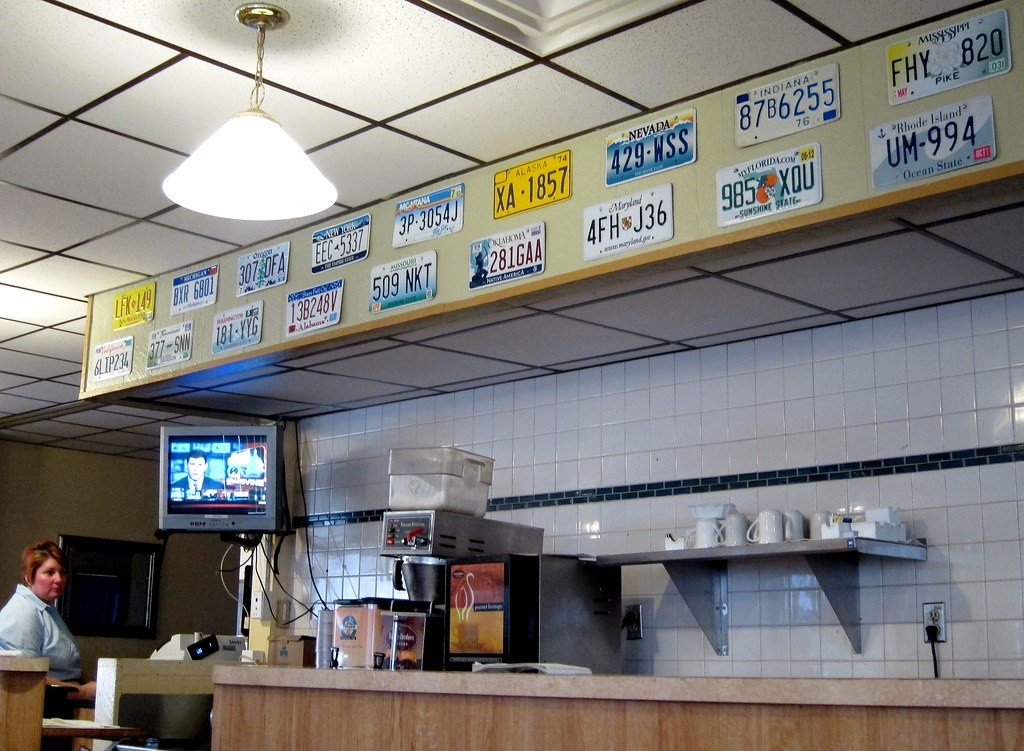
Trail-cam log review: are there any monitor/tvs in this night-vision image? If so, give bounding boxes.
[157,427,286,530]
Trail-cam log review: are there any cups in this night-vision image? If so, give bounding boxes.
[810,511,830,539]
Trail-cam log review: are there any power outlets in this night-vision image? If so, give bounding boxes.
[922,602,946,643]
[626,604,643,640]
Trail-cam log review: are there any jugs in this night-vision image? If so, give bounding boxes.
[782,510,803,541]
[694,519,723,548]
[714,514,748,546]
[747,510,782,544]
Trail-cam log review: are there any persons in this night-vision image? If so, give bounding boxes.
[171,449,224,499]
[0,538,97,751]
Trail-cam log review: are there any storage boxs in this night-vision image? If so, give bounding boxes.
[267,635,316,667]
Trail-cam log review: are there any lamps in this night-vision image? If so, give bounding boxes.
[163,3,338,220]
